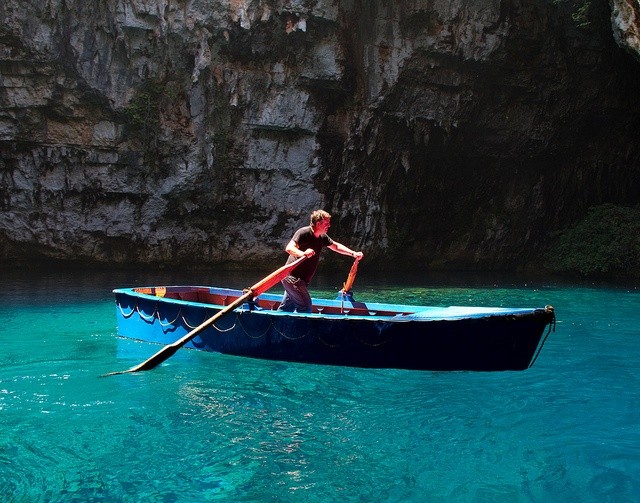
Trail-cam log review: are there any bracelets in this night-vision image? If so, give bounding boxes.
[351,250,355,257]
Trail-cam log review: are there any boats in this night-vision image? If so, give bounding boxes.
[97,252,554,376]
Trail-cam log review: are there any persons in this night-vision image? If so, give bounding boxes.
[278,208,363,312]
